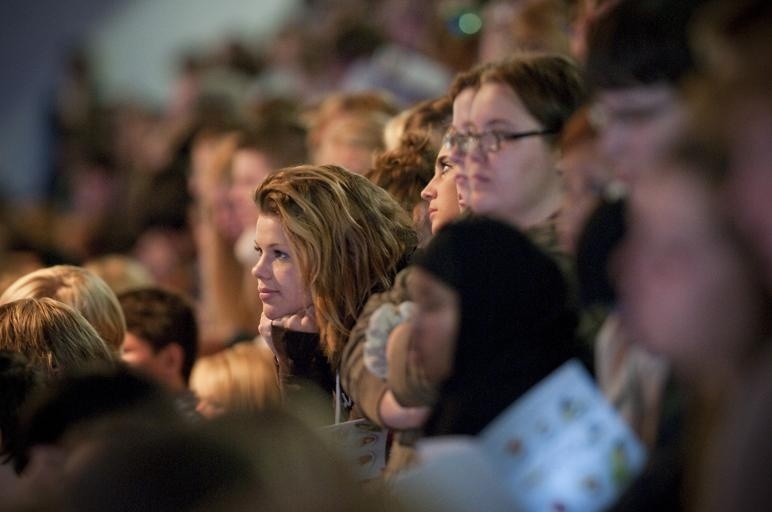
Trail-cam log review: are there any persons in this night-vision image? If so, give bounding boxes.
[0,1,772,511]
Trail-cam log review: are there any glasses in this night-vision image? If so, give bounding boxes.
[463,125,561,152]
